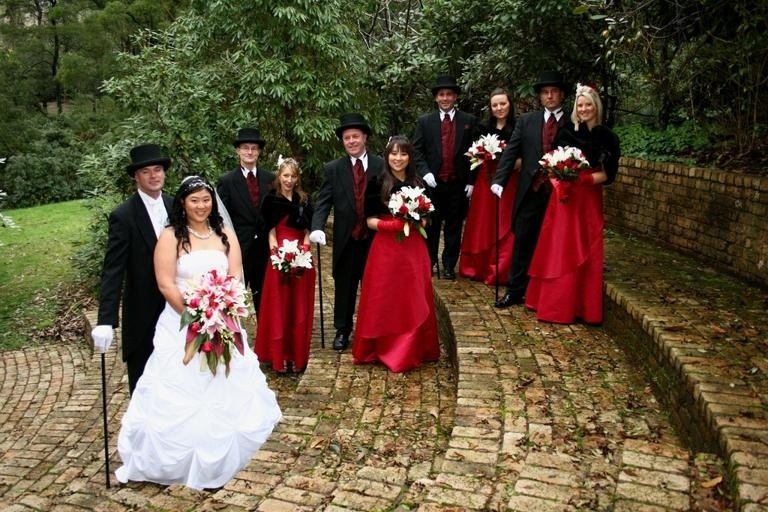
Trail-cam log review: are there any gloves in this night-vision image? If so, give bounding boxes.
[423,173,437,188]
[491,184,503,198]
[91,324,114,353]
[309,230,326,246]
[465,185,474,197]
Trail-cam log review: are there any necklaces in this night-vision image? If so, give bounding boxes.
[188,227,213,241]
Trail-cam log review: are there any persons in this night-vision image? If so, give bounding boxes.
[308,113,385,350]
[114,175,285,492]
[216,128,276,323]
[254,154,314,373]
[351,136,441,373]
[489,71,573,309]
[460,87,522,287]
[90,144,176,400]
[411,75,479,280]
[524,83,620,324]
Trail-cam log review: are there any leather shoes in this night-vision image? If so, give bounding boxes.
[333,331,349,350]
[494,293,521,307]
[443,268,456,279]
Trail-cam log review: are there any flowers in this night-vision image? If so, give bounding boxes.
[387,185,435,241]
[463,132,508,181]
[538,146,591,201]
[180,270,258,378]
[270,239,313,280]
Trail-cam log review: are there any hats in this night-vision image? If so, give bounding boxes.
[126,144,171,178]
[533,71,568,91]
[335,113,370,140]
[232,128,266,149]
[431,76,461,97]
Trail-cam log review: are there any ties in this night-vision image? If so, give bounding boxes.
[545,113,556,153]
[442,113,454,182]
[247,171,259,208]
[353,160,365,239]
